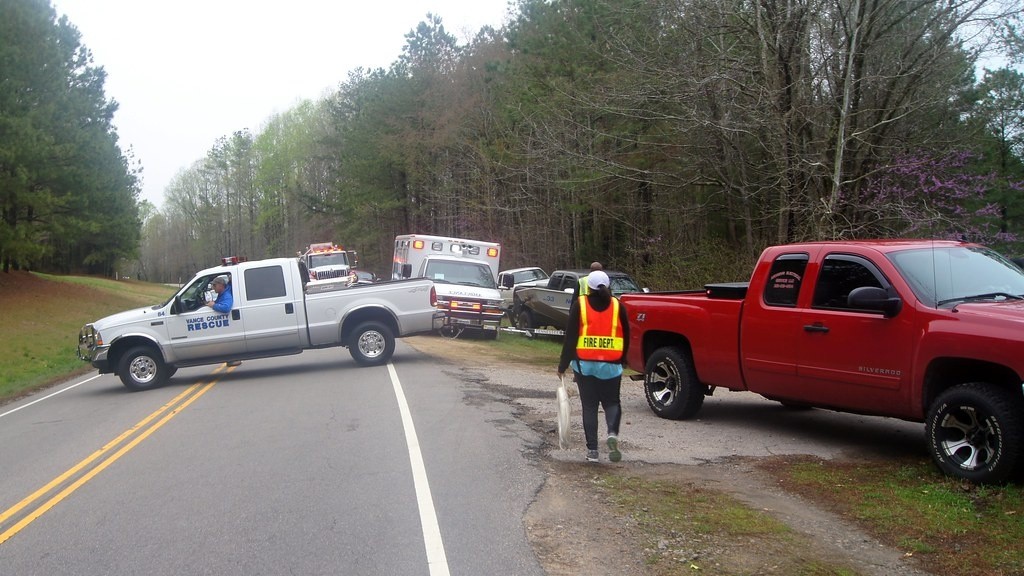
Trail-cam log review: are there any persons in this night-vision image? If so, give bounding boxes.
[557,269,630,462]
[205,276,233,313]
[568,261,603,312]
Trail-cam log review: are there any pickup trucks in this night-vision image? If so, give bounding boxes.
[77,256,446,391]
[619,240,1024,482]
[514,270,641,340]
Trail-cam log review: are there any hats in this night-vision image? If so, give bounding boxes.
[209,277,226,286]
[587,270,610,290]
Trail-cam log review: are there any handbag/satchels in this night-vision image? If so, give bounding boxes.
[556,374,572,451]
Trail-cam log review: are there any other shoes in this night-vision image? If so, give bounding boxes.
[606,433,622,463]
[586,450,599,462]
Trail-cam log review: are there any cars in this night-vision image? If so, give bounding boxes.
[497,267,550,309]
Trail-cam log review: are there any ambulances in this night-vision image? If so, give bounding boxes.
[391,235,506,340]
[298,242,358,280]
